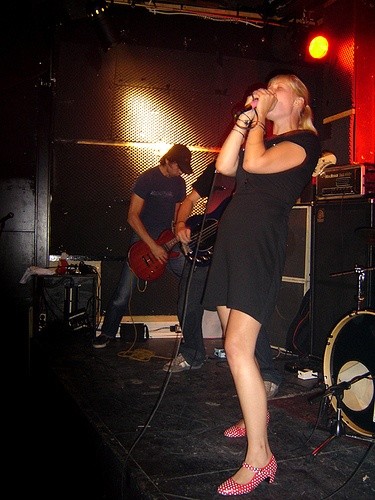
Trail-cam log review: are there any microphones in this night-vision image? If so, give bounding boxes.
[234,98,257,117]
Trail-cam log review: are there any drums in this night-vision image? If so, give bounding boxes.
[324,310,375,438]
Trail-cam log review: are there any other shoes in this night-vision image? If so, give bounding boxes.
[262,380,281,400]
[163,352,203,371]
[92,335,113,348]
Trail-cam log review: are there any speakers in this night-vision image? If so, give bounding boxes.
[310,195,375,364]
[262,204,309,354]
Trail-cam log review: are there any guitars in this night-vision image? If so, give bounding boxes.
[129,228,179,281]
[181,152,336,266]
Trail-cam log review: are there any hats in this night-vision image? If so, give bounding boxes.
[169,143,194,174]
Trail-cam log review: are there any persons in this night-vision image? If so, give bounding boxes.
[164,159,283,399]
[90,144,194,349]
[215,75,325,496]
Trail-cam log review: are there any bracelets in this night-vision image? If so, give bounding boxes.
[176,221,186,226]
[238,117,250,126]
[251,120,265,130]
[232,128,245,138]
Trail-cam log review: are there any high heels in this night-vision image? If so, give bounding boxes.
[218,454,277,495]
[223,408,270,438]
